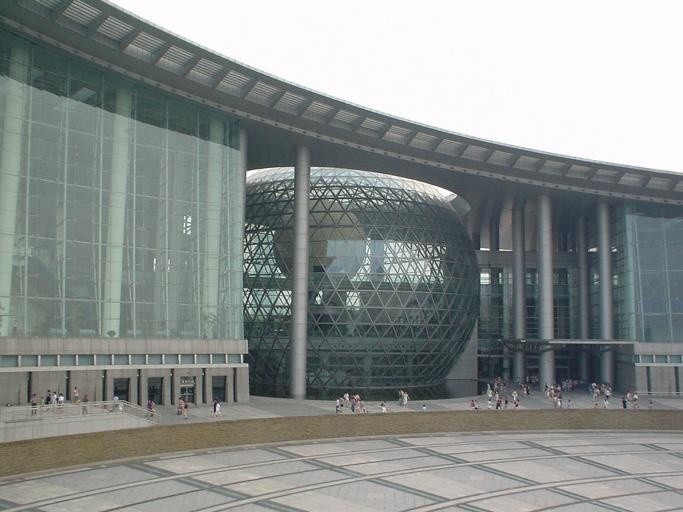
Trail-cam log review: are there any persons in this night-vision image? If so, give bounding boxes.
[30,386,89,417]
[176,396,189,419]
[212,398,222,418]
[113,394,119,409]
[335,391,365,414]
[398,389,410,407]
[380,403,387,412]
[470,373,640,410]
[384,401,387,407]
[144,400,156,417]
[422,404,426,410]
[649,400,653,409]
